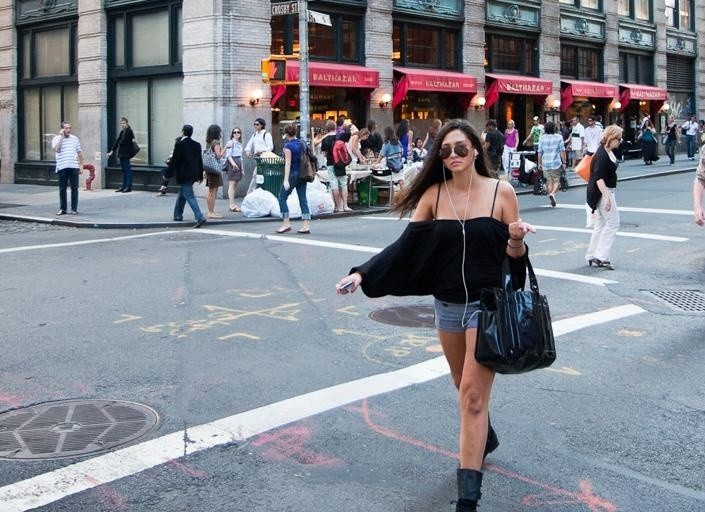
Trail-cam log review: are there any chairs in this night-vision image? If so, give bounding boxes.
[367,167,394,211]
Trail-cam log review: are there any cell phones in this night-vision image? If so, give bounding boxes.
[341,281,352,292]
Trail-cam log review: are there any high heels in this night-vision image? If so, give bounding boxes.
[589,259,614,270]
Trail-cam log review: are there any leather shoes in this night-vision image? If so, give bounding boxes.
[276,227,290,233]
[297,229,310,234]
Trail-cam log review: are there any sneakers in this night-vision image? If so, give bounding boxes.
[116,187,133,193]
[193,219,206,228]
[549,193,557,207]
[57,209,78,215]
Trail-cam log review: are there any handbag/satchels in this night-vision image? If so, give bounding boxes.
[681,128,690,134]
[202,141,222,174]
[299,141,319,183]
[474,255,556,374]
[575,144,602,181]
[534,170,547,195]
[131,142,139,157]
[662,135,668,144]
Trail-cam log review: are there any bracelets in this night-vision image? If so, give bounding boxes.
[507,236,525,248]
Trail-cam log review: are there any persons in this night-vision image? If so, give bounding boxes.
[336,119,537,512]
[52,121,83,215]
[105,117,135,192]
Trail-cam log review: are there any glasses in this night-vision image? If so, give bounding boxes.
[254,122,259,125]
[437,145,475,158]
[232,131,240,134]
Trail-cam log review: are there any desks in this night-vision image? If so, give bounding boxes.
[346,170,371,203]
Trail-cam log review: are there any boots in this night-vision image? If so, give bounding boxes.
[454,468,483,512]
[481,413,499,461]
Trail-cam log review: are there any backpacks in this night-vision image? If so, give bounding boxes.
[332,139,352,168]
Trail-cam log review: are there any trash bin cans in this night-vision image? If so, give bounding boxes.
[254,155,285,199]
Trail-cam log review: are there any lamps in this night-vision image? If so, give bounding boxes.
[248,89,262,106]
[612,101,622,111]
[474,97,487,110]
[551,99,561,111]
[380,93,391,108]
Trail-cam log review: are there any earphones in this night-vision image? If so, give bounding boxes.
[474,149,478,157]
